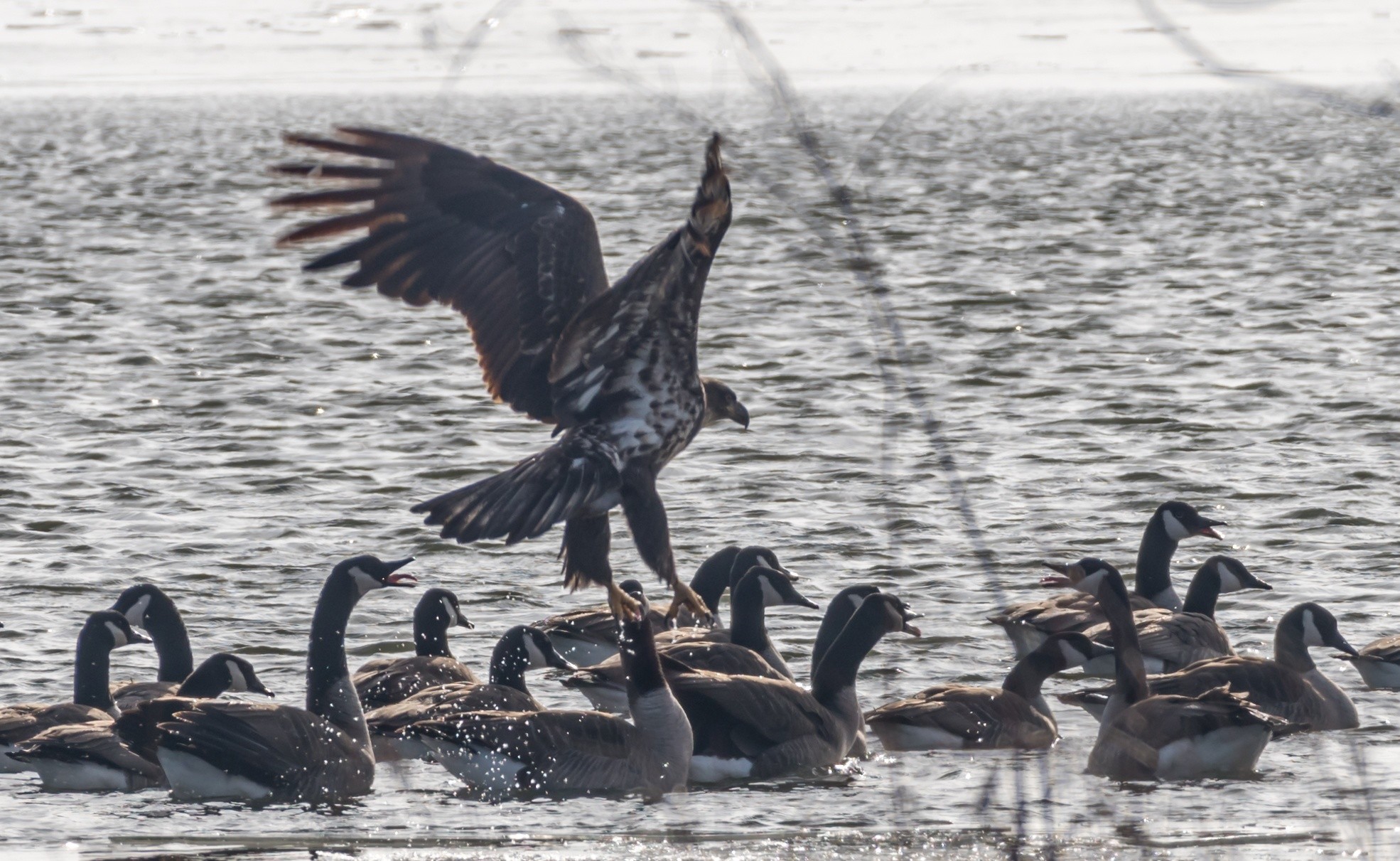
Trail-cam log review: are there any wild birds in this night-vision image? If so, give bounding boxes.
[350,587,482,742]
[350,543,927,797]
[0,556,420,806]
[860,501,1400,785]
[258,125,748,628]
